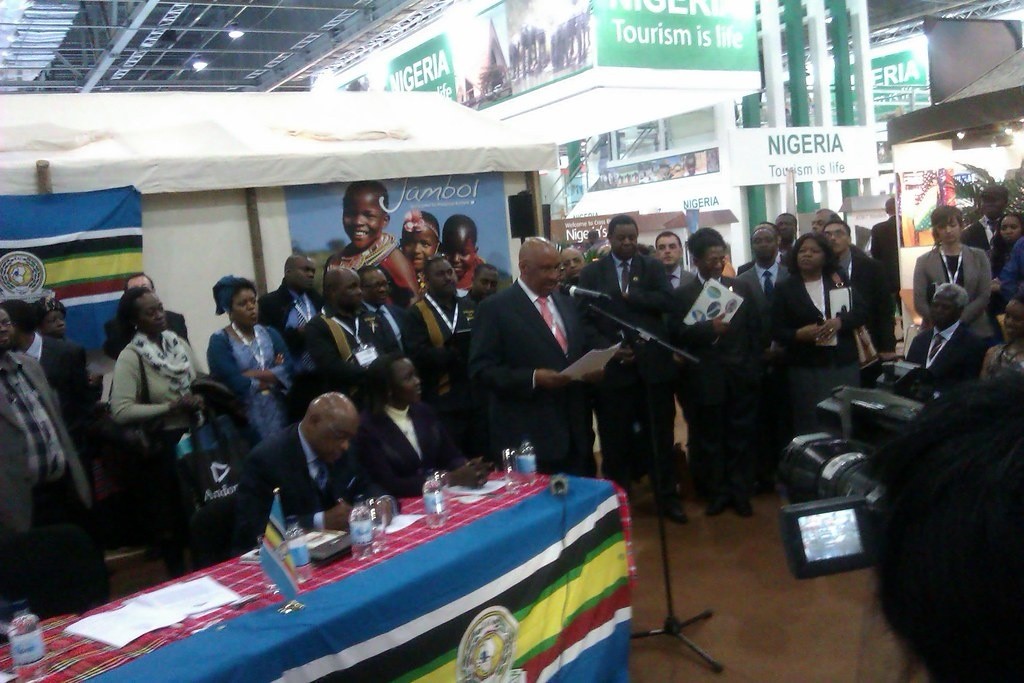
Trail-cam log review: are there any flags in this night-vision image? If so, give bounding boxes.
[0,186,143,358]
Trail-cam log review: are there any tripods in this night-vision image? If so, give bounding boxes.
[587,300,724,673]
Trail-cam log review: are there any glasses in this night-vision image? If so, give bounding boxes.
[524,260,566,276]
[360,281,391,289]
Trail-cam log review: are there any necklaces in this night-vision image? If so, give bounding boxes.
[941,245,963,285]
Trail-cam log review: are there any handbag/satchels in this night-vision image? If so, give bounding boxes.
[829,271,879,370]
[90,349,150,502]
[182,402,251,505]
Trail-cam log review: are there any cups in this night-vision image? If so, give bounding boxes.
[432,470,449,515]
[365,498,385,552]
[503,449,520,493]
[257,535,278,590]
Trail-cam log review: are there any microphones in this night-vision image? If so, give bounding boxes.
[569,285,610,300]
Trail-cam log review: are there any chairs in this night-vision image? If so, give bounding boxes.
[187,497,235,573]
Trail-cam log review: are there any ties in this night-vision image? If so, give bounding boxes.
[928,334,944,361]
[619,262,630,293]
[296,299,313,369]
[314,458,329,495]
[667,274,675,289]
[374,308,402,351]
[537,296,568,359]
[763,270,774,297]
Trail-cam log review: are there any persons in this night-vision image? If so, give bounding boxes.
[442,214,487,306]
[400,210,439,303]
[355,351,493,504]
[907,186,1024,407]
[227,391,369,555]
[0,296,110,621]
[322,180,419,310]
[868,370,1024,683]
[101,210,895,579]
[870,198,904,344]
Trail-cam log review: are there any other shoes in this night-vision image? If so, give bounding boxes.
[164,546,185,577]
[688,466,775,518]
[657,497,685,525]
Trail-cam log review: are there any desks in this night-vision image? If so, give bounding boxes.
[0,470,635,683]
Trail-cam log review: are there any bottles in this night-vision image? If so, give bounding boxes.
[350,495,372,560]
[286,516,314,582]
[516,436,536,485]
[9,600,45,683]
[423,470,446,529]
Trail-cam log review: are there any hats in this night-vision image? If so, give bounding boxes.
[36,296,66,320]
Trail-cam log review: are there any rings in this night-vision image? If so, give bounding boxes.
[466,462,473,467]
[829,328,833,332]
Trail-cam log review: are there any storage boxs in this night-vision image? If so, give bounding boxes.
[106,541,167,603]
